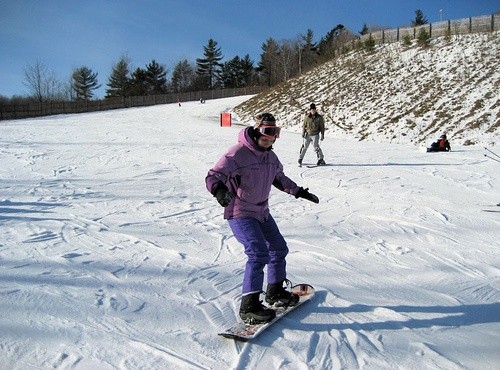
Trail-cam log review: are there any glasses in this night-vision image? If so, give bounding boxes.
[260,126,281,138]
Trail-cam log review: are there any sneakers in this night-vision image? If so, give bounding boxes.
[239,291,276,324]
[265,282,299,306]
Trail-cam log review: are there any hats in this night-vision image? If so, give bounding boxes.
[310,103,316,110]
[255,113,276,130]
[440,135,446,138]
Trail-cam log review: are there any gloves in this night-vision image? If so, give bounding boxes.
[217,188,231,207]
[300,188,319,203]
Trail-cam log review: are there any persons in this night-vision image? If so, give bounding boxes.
[428,135,451,153]
[299,104,327,168]
[207,114,318,323]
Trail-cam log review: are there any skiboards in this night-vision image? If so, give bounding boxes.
[297,162,326,168]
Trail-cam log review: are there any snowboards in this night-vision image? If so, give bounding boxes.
[217,283,316,342]
